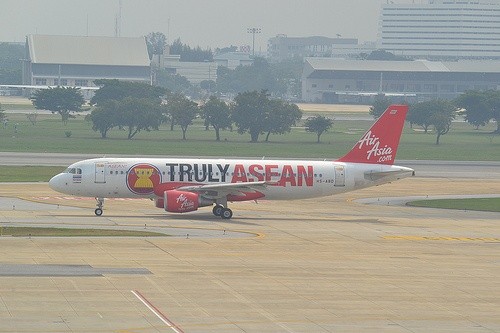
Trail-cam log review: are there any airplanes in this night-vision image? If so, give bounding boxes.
[48,103,416,220]
